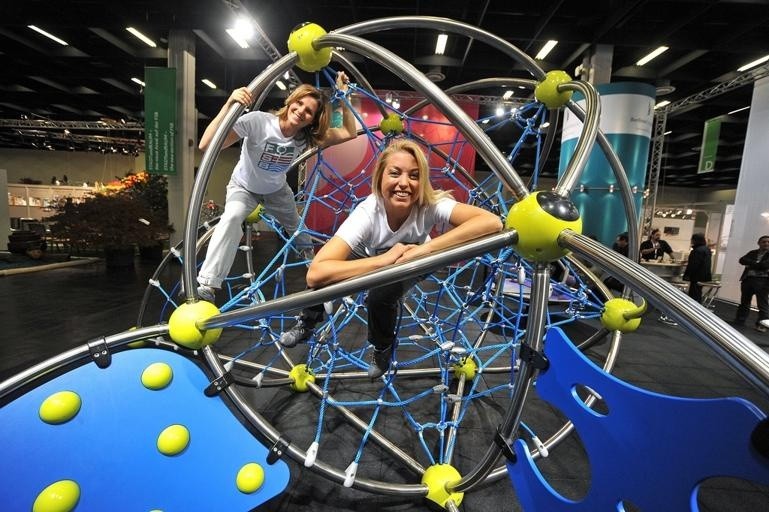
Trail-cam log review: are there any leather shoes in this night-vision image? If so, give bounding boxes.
[729,319,743,325]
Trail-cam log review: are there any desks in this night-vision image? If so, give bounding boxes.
[490,278,588,331]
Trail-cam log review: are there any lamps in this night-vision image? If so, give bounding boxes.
[29,139,140,158]
[656,209,692,218]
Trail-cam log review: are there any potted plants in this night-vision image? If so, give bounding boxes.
[41,172,169,269]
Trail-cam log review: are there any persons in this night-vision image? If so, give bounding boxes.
[592,233,629,303]
[277,140,504,382]
[682,232,714,303]
[174,67,357,303]
[638,228,676,263]
[730,235,769,334]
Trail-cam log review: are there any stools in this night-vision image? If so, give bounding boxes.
[697,280,721,309]
[658,281,688,326]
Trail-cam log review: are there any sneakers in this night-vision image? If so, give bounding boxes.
[367,343,393,378]
[304,246,315,268]
[178,288,216,304]
[279,320,315,348]
